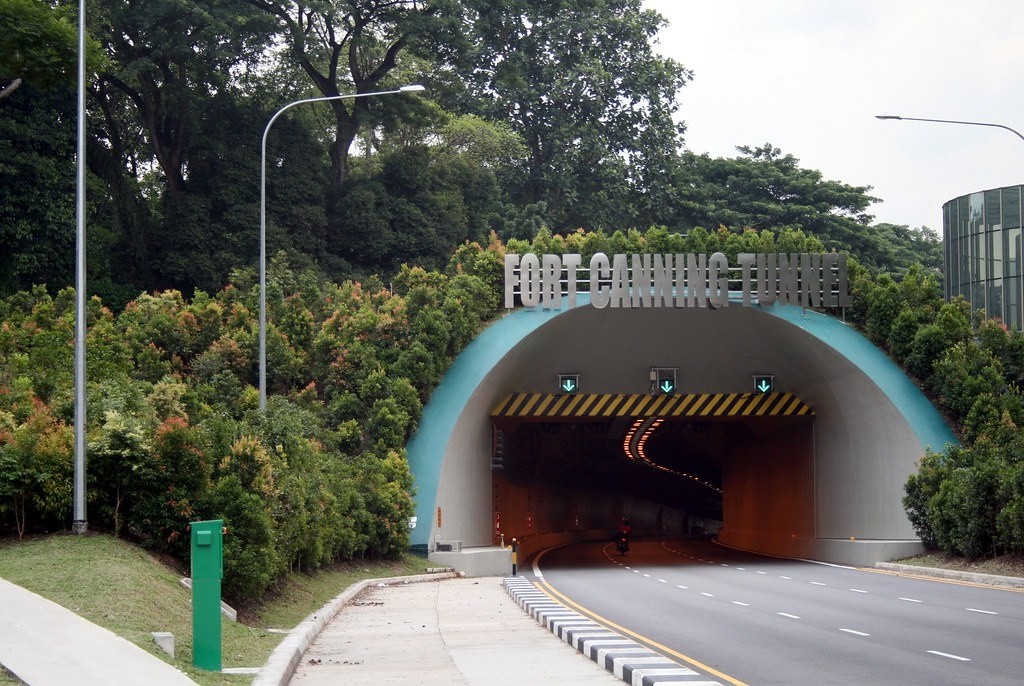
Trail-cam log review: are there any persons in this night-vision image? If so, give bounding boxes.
[614,518,632,551]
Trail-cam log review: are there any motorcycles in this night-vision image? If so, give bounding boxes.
[616,525,630,555]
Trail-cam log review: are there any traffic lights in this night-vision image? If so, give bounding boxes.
[557,375,580,393]
[754,375,774,393]
[650,366,680,395]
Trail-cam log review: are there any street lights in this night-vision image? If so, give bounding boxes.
[260,84,425,413]
[876,115,1024,144]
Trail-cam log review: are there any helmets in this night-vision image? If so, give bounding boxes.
[623,517,629,524]
